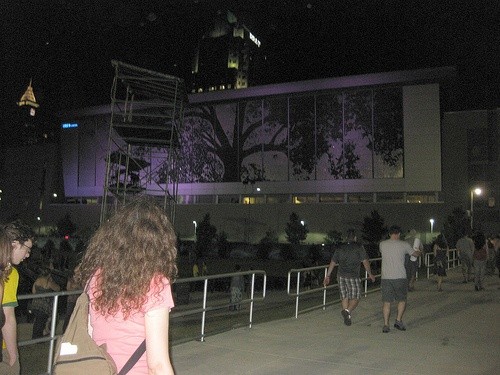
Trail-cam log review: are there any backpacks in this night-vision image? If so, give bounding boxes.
[51,291,146,375]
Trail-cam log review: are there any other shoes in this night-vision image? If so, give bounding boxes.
[383,326,390,333]
[393,321,406,330]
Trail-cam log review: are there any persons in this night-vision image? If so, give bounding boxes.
[0,219,83,375]
[379,226,421,333]
[230,265,244,310]
[324,229,375,326]
[52,195,178,375]
[403,227,500,291]
[303,265,315,290]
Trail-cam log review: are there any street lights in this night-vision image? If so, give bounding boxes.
[470,186,482,229]
[429,219,434,233]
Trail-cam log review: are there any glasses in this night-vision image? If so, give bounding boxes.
[23,245,32,255]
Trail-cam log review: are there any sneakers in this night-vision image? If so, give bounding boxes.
[343,309,352,326]
